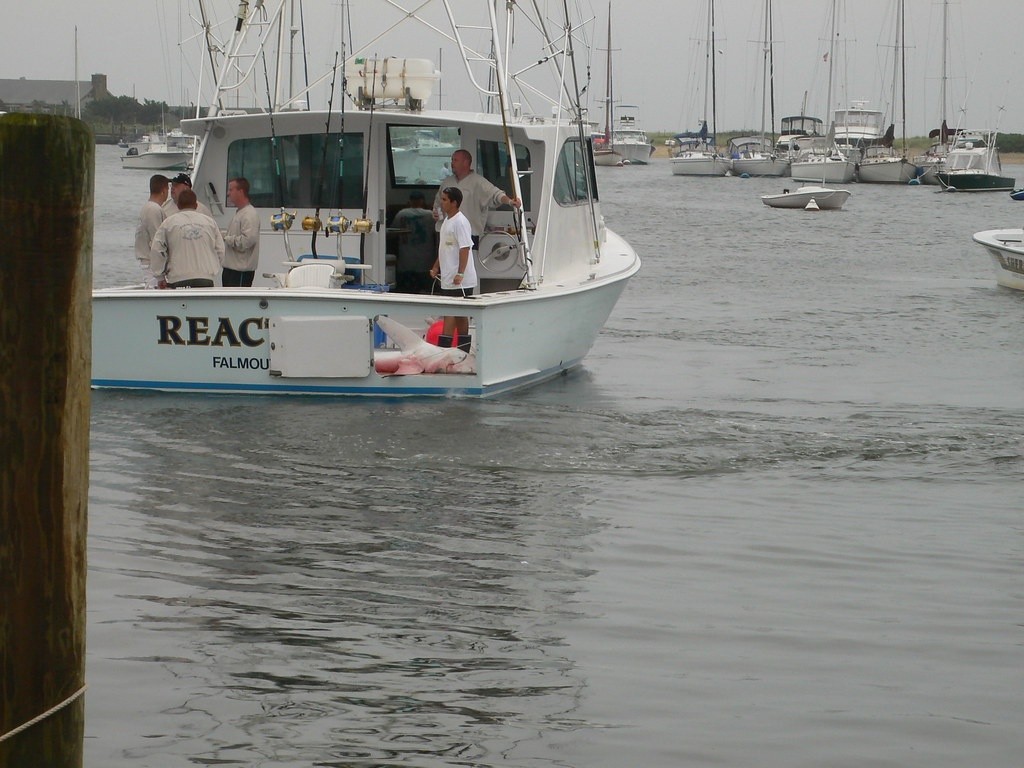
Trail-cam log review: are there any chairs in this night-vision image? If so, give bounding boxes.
[264,263,353,291]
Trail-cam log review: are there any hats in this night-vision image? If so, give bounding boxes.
[409,190,426,200]
[168,172,192,188]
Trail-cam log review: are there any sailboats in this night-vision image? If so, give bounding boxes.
[760,0,851,213]
[121,1,1024,293]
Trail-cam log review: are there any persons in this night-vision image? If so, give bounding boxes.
[439,163,451,180]
[429,187,478,354]
[149,190,226,288]
[433,149,522,294]
[221,177,261,287]
[136,174,171,291]
[390,191,435,289]
[160,172,213,219]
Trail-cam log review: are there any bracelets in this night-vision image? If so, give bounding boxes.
[457,272,464,278]
[508,199,512,207]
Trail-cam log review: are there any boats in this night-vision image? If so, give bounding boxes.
[88,2,646,406]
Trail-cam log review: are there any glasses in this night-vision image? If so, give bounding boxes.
[445,187,455,196]
